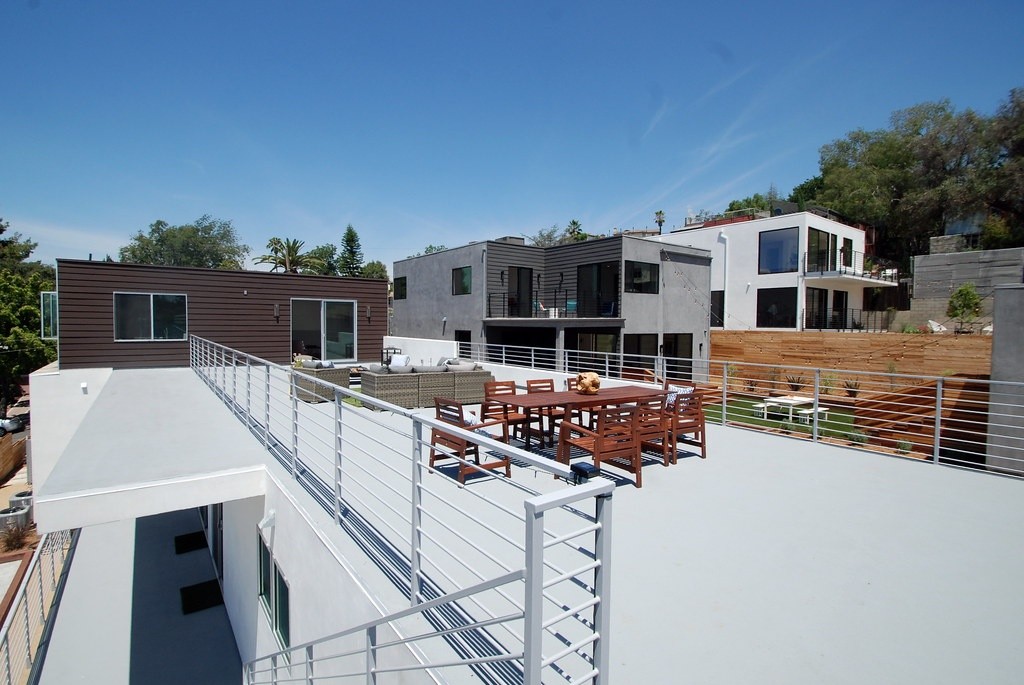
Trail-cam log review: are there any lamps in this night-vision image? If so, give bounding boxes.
[273,304,281,318]
[366,305,371,318]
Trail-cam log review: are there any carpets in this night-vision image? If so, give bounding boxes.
[174,530,209,555]
[341,387,367,407]
[180,578,225,616]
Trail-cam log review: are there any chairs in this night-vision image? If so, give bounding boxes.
[291,355,320,362]
[380,347,402,367]
[534,299,577,319]
[429,377,706,489]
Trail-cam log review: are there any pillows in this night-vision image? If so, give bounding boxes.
[439,405,493,439]
[665,384,695,414]
[303,360,335,369]
[370,357,477,374]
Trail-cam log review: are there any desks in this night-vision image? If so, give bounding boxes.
[486,385,678,466]
[334,363,381,385]
[764,395,816,424]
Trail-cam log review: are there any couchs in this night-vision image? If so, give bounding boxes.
[291,364,351,404]
[360,356,496,411]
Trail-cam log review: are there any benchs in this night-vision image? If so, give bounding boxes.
[752,402,830,425]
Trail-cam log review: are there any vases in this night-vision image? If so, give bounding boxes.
[0,489,33,531]
